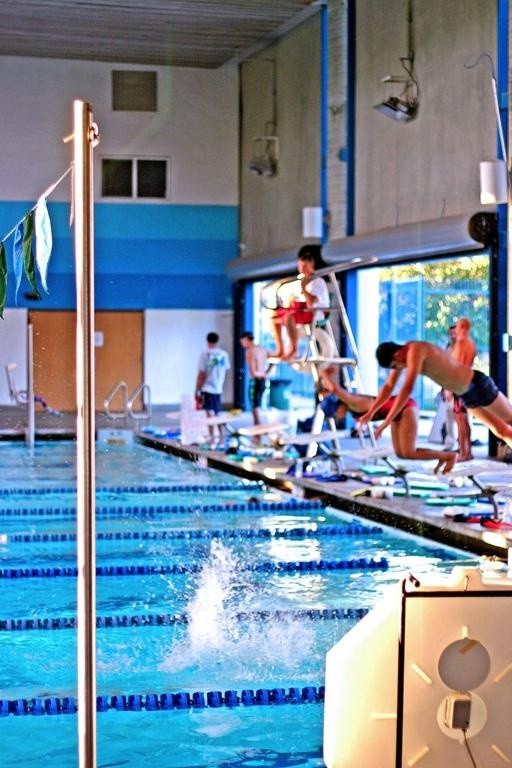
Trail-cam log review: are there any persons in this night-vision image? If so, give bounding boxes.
[359,340,512,451]
[195,333,231,445]
[427,325,461,453]
[447,317,476,463]
[316,366,459,475]
[238,333,273,444]
[267,253,330,360]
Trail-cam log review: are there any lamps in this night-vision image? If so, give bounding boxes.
[244,31,288,183]
[301,203,327,238]
[372,5,427,132]
[476,154,507,208]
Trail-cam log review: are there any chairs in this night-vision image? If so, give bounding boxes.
[302,291,335,360]
[3,363,28,425]
